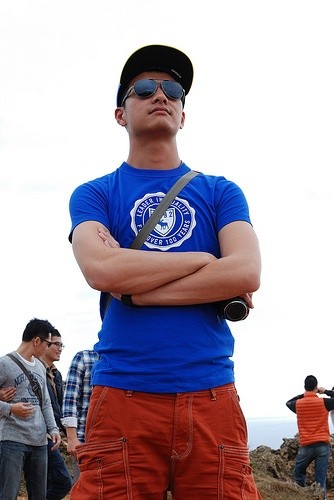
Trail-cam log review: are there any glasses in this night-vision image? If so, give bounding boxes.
[121,79,185,105]
[43,339,52,347]
[52,343,64,348]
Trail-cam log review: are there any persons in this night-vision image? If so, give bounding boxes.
[286,375,334,500]
[68,43,261,500]
[0,318,99,500]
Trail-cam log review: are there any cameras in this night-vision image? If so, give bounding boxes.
[215,296,249,321]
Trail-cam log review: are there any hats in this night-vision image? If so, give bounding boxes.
[116,45,194,106]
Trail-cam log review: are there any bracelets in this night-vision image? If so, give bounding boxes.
[121,294,134,307]
